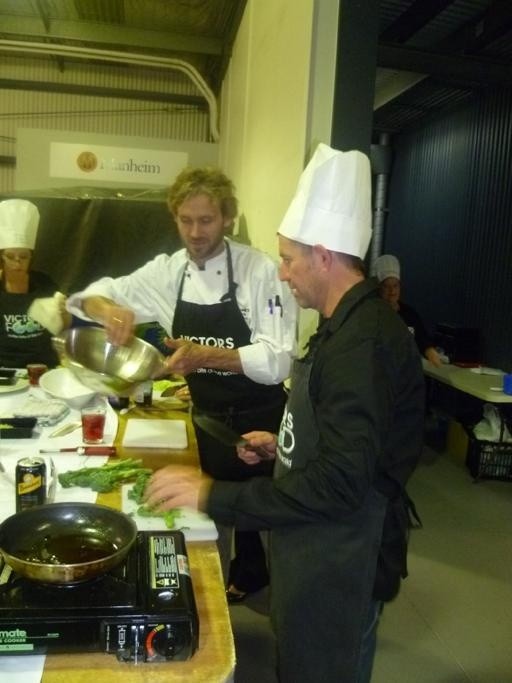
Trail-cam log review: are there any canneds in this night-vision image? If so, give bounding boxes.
[16,457,45,515]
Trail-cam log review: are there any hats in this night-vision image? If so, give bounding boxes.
[277,141,374,262]
[378,252,401,282]
[1,199,41,252]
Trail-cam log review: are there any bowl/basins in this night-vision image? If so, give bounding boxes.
[39,367,100,409]
[58,326,169,396]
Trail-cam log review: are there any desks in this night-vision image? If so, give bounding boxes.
[420,358,512,474]
[0,363,242,680]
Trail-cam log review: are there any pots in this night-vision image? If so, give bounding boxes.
[0,500,140,588]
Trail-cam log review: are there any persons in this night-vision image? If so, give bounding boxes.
[0,249,72,371]
[380,276,441,368]
[142,232,427,680]
[65,167,301,606]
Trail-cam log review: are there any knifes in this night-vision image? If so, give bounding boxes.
[192,415,272,460]
[36,443,119,458]
[160,383,190,397]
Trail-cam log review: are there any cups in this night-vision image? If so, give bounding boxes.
[133,380,153,409]
[79,407,108,443]
[108,393,129,410]
[26,364,48,386]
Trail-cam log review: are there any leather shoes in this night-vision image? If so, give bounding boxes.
[225,585,250,604]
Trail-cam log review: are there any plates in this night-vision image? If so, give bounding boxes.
[0,366,31,394]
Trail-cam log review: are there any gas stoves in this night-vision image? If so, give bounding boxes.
[0,530,202,665]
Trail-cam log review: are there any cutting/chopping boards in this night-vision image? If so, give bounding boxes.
[121,473,220,544]
[121,415,189,449]
